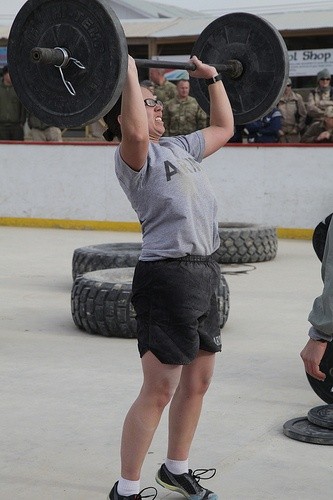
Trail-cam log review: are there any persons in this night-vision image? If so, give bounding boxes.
[102,53,235,500]
[299,214,333,380]
[0,65,333,144]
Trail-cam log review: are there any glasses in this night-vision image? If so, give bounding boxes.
[320,78,330,81]
[285,84,291,88]
[144,98,163,106]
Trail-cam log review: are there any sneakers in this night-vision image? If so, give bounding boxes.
[155,462,219,500]
[105,480,157,500]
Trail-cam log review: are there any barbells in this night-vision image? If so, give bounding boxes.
[5,0,286,129]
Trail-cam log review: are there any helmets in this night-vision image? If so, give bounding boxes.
[317,69,331,82]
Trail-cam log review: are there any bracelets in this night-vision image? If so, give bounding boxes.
[206,74,222,86]
